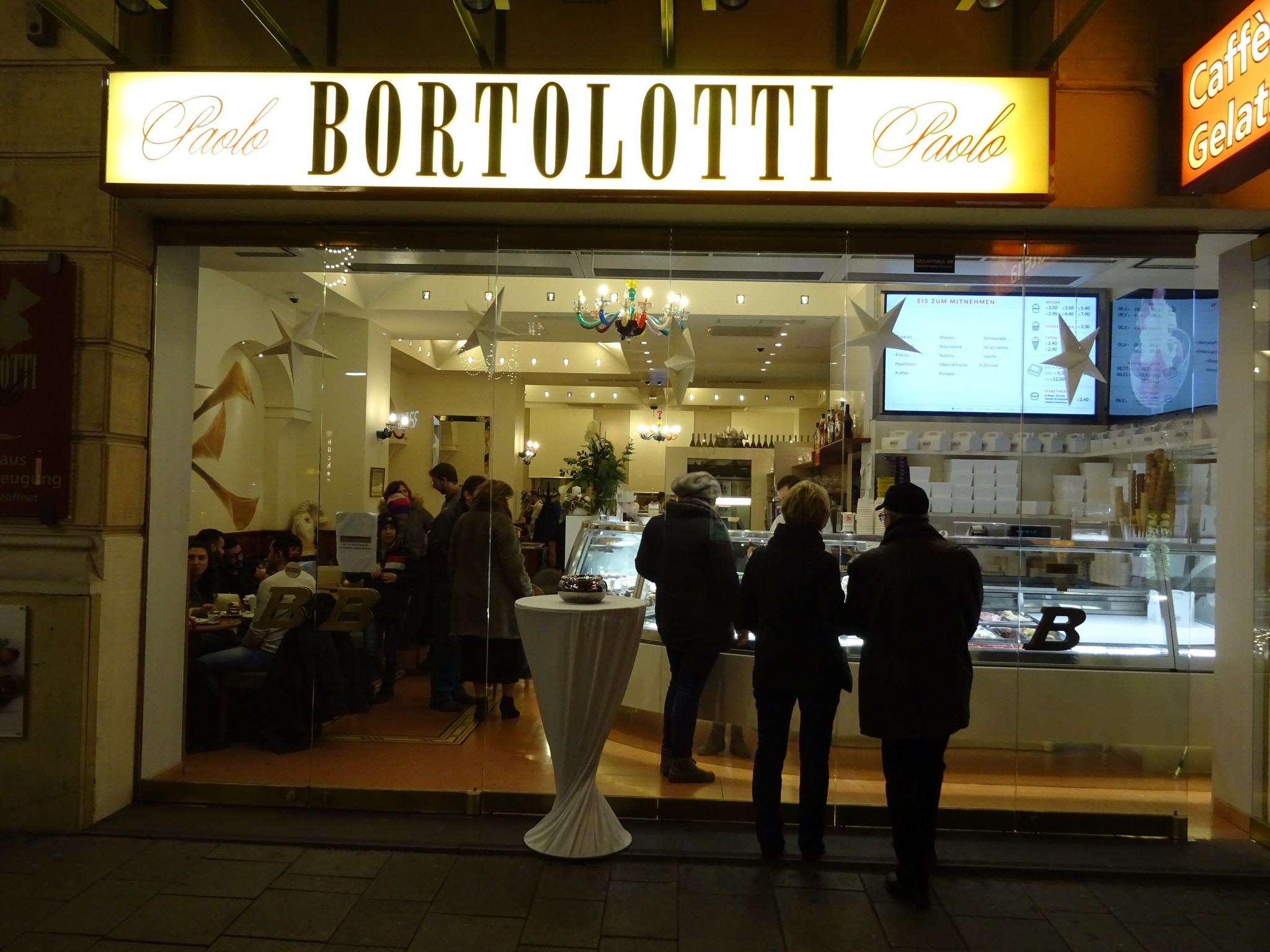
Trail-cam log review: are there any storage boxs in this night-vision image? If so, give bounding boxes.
[881,417,1209,454]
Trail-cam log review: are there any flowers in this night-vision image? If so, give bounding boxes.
[558,420,637,516]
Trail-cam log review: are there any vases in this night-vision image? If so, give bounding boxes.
[590,499,617,521]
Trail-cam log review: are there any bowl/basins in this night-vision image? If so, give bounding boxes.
[558,574,607,603]
[856,497,887,535]
[688,464,751,477]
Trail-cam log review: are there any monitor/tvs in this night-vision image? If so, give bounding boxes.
[873,285,1111,432]
[1111,288,1220,425]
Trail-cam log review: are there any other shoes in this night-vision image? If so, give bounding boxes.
[455,695,475,707]
[885,880,932,909]
[429,698,464,714]
[668,757,715,784]
[660,746,676,777]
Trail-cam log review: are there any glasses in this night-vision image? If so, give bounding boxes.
[878,512,893,523]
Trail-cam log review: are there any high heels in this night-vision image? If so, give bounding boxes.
[499,695,520,721]
[473,697,489,721]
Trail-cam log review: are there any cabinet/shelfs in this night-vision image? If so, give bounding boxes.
[869,418,1218,520]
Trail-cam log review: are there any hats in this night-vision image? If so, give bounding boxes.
[875,482,929,515]
[670,471,722,500]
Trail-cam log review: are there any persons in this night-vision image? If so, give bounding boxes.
[186,528,315,752]
[635,471,750,781]
[844,481,985,904]
[348,462,544,713]
[446,479,533,720]
[736,477,844,858]
[523,490,566,573]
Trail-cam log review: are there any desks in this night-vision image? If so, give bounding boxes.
[188,618,242,667]
[514,595,647,860]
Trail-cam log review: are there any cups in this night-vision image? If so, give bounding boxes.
[244,597,257,613]
[648,504,659,517]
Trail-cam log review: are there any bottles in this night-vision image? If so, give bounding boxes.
[207,611,220,623]
[689,428,810,449]
[812,401,863,451]
[572,486,581,498]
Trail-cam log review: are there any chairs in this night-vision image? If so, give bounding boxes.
[212,565,345,754]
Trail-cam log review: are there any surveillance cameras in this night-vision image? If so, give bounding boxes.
[644,381,650,385]
[756,344,766,352]
[657,381,662,385]
[286,294,299,303]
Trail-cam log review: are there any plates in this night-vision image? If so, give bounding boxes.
[221,611,242,617]
[1089,552,1131,586]
[242,611,254,618]
[195,619,219,624]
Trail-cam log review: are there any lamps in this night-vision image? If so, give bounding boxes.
[572,279,689,344]
[518,440,539,465]
[637,411,681,443]
[376,413,409,439]
